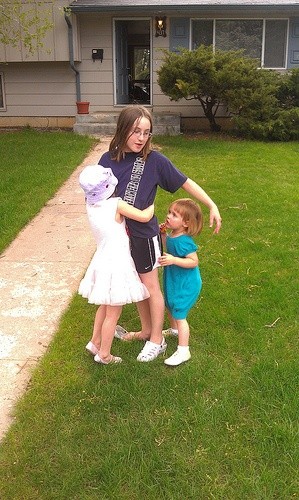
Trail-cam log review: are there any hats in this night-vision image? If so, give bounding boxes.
[79,166,118,205]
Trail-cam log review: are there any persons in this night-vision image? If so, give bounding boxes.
[98,104,222,360]
[78,165,154,365]
[157,199,204,366]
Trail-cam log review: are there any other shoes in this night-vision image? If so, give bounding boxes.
[94,354,122,364]
[164,345,191,366]
[85,341,101,356]
[161,328,179,336]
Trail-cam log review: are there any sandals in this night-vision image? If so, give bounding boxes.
[137,336,167,362]
[122,331,138,342]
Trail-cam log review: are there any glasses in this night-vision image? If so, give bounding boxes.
[130,130,152,137]
